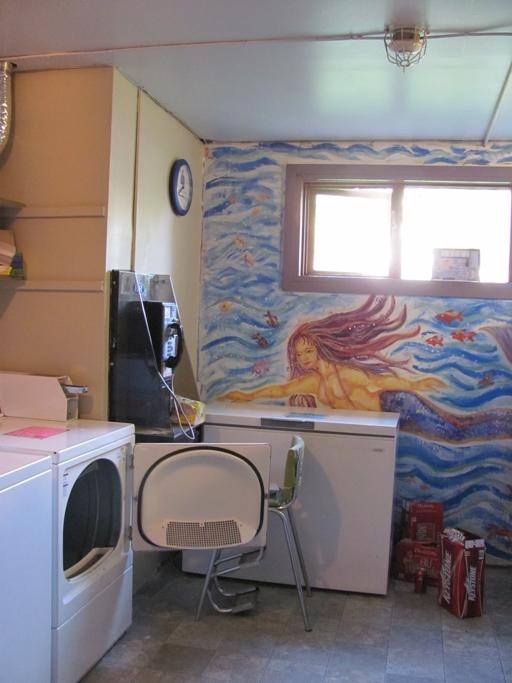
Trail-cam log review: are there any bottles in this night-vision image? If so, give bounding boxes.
[415,568,427,594]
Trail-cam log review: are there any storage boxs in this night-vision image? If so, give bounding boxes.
[400,498,443,538]
[435,524,487,621]
[395,537,442,589]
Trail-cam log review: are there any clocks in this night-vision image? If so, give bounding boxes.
[169,159,193,217]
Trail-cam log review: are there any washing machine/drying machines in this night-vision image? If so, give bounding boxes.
[0,415,273,683]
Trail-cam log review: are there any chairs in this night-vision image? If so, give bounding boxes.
[196,436,313,632]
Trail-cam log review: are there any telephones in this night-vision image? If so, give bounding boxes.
[115,302,185,425]
